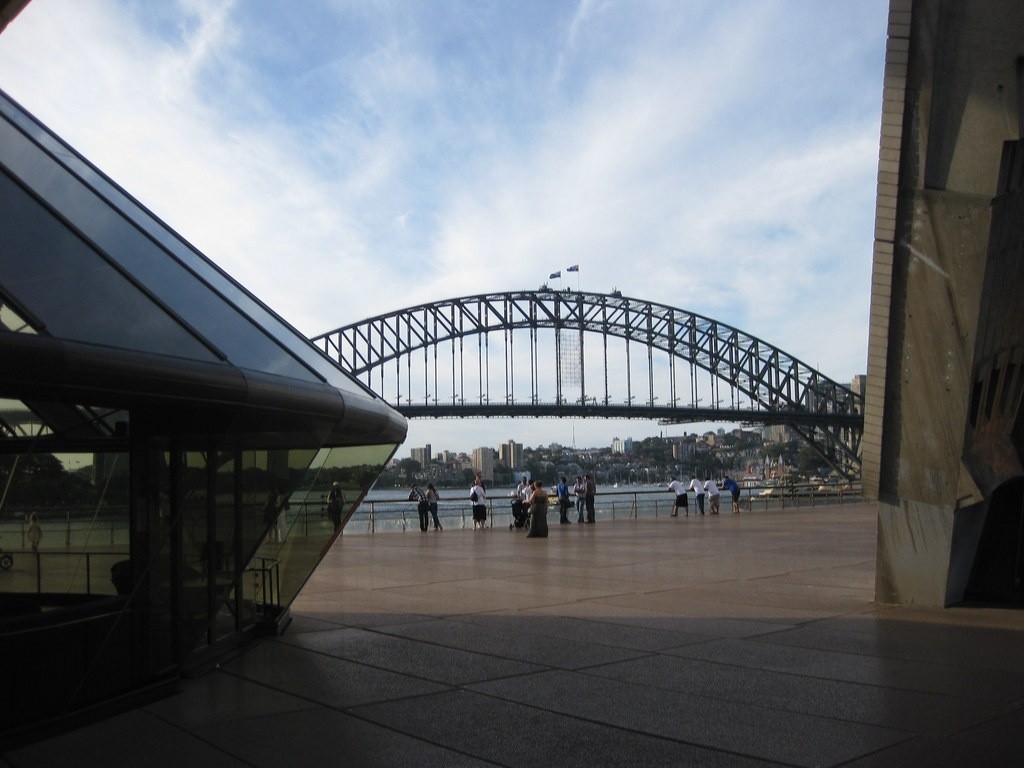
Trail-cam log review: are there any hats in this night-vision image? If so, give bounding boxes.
[333,481,339,486]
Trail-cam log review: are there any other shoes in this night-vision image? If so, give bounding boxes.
[710,512,719,514]
[432,528,438,532]
[584,520,595,524]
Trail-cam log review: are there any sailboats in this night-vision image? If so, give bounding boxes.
[613,467,686,488]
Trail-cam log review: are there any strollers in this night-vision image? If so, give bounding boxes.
[509,498,531,531]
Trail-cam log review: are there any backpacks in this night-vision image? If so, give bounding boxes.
[471,486,481,503]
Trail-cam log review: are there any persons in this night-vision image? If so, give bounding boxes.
[721,476,741,513]
[686,475,705,515]
[523,480,548,538]
[703,477,720,514]
[556,477,571,523]
[327,481,344,536]
[517,477,529,501]
[263,489,278,545]
[409,484,429,531]
[668,476,689,517]
[425,483,443,531]
[470,474,486,493]
[521,478,536,503]
[28,512,42,551]
[573,476,586,523]
[583,474,597,523]
[275,491,287,544]
[470,479,488,530]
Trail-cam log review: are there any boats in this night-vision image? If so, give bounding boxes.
[751,478,860,498]
[548,486,575,508]
